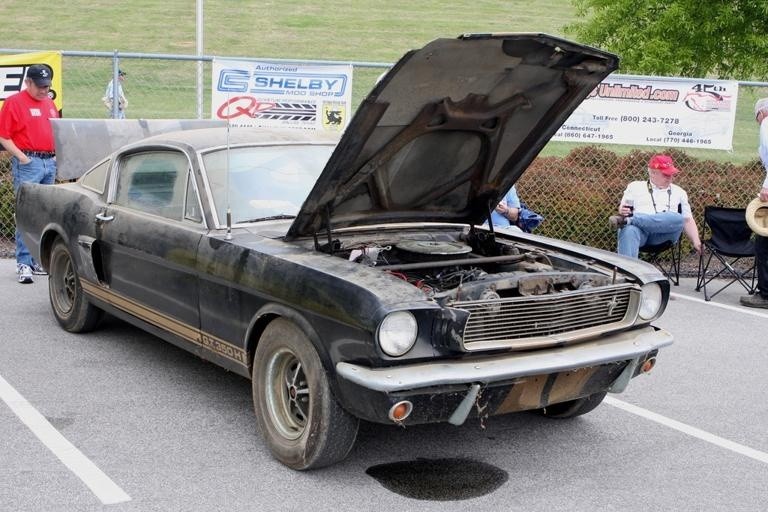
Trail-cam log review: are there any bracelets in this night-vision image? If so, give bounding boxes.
[503,207,509,216]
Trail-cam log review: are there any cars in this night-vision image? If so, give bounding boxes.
[8,23,679,481]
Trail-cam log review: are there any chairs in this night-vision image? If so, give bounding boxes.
[695,204,759,301]
[638,203,683,286]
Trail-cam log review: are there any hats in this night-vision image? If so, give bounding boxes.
[649,155,680,175]
[28,64,54,87]
[745,196,768,236]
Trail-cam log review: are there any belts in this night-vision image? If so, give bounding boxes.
[22,150,56,158]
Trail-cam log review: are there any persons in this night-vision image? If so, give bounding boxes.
[609,155,704,259]
[105,70,128,119]
[0,63,60,284]
[739,98,768,308]
[485,185,522,229]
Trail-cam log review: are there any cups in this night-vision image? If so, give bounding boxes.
[624,198,635,218]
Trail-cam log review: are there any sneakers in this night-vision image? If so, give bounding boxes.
[740,292,768,308]
[16,263,46,283]
[608,215,625,231]
